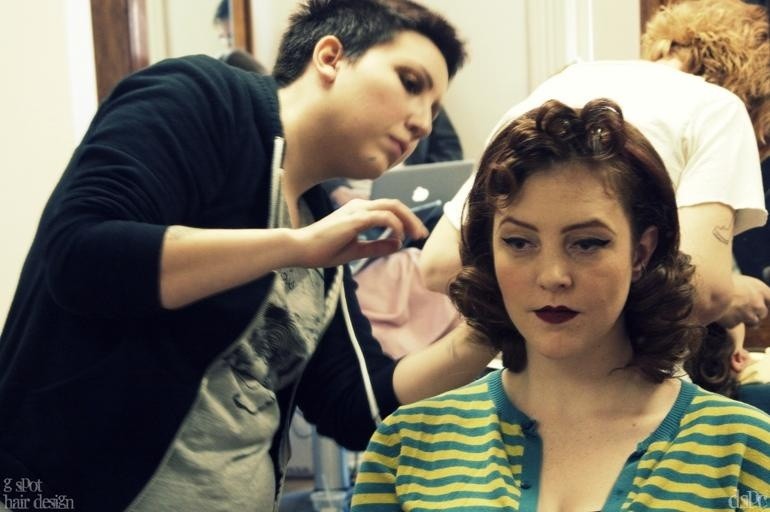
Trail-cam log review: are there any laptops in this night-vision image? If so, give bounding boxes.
[369,159,479,214]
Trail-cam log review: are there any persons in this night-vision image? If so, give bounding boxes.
[417,0,769,334]
[693,316,769,411]
[210,2,231,54]
[320,95,463,215]
[343,97,769,512]
[2,1,505,512]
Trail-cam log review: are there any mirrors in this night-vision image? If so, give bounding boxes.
[90,0,252,114]
